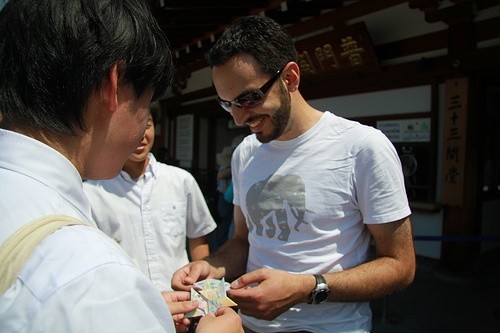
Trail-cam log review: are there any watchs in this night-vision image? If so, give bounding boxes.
[307,273,331,305]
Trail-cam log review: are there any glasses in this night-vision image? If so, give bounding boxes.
[217,68,285,112]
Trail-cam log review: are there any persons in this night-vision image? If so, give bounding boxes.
[0,0,246,332]
[170,8,418,333]
[81,108,217,332]
